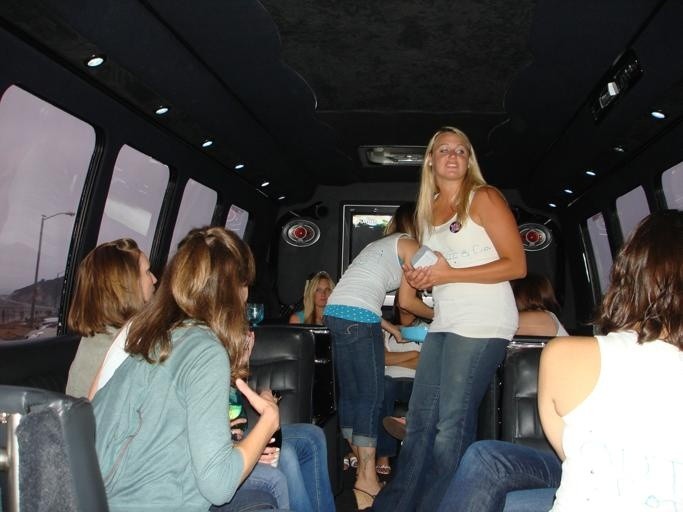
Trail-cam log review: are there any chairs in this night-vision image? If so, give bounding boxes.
[1,321,611,512]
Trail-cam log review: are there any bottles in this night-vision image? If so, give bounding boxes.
[257,420,281,469]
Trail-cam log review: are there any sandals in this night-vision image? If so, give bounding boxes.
[342,450,393,477]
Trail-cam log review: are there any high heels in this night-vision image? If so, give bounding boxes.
[353,484,378,511]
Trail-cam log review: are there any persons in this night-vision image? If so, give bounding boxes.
[77,222,286,511]
[60,236,312,512]
[431,434,564,512]
[241,420,338,512]
[357,121,530,512]
[320,199,435,510]
[380,265,573,443]
[534,206,681,511]
[287,269,360,471]
[372,286,430,476]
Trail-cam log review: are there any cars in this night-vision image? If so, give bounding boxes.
[23,317,58,340]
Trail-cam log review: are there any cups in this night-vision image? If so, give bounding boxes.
[246,303,265,327]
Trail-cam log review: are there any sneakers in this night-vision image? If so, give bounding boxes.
[381,413,407,443]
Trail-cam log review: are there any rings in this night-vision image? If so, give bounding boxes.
[230,429,234,435]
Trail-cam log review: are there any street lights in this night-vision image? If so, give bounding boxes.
[29,210,75,328]
